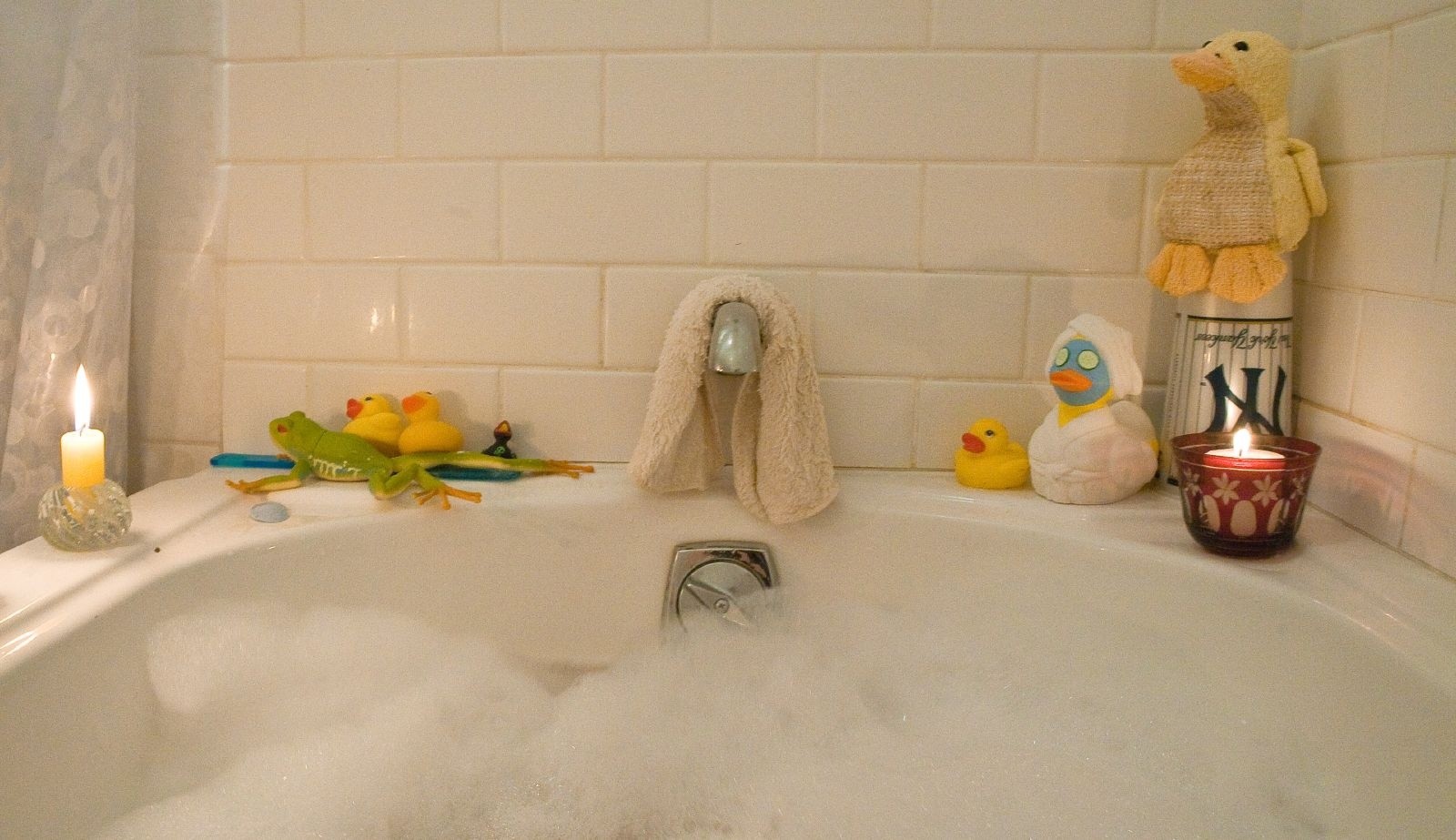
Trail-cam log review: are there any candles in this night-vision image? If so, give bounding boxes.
[60,364,106,491]
[1202,429,1285,460]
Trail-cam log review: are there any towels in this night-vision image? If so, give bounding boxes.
[626,274,840,525]
[1042,314,1142,400]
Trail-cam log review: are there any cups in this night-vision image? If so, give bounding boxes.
[1169,433,1323,560]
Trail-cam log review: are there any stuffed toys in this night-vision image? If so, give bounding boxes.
[1147,27,1327,303]
[1028,313,1160,506]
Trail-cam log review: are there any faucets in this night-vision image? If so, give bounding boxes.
[709,303,763,377]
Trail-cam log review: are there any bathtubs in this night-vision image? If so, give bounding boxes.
[0,458,1455,840]
